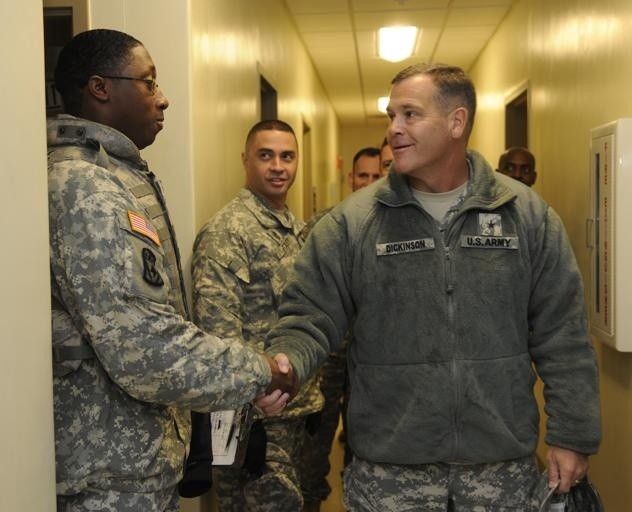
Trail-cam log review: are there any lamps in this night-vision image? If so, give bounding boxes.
[373,22,423,63]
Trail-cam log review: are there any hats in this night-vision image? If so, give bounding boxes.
[530,463,604,512]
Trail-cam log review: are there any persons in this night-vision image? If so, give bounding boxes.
[302,137,395,511]
[254,63,602,512]
[495,147,536,187]
[191,121,325,511]
[48,29,300,512]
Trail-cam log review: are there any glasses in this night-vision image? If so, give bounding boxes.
[99,75,159,93]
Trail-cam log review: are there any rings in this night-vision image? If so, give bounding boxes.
[575,480,582,484]
